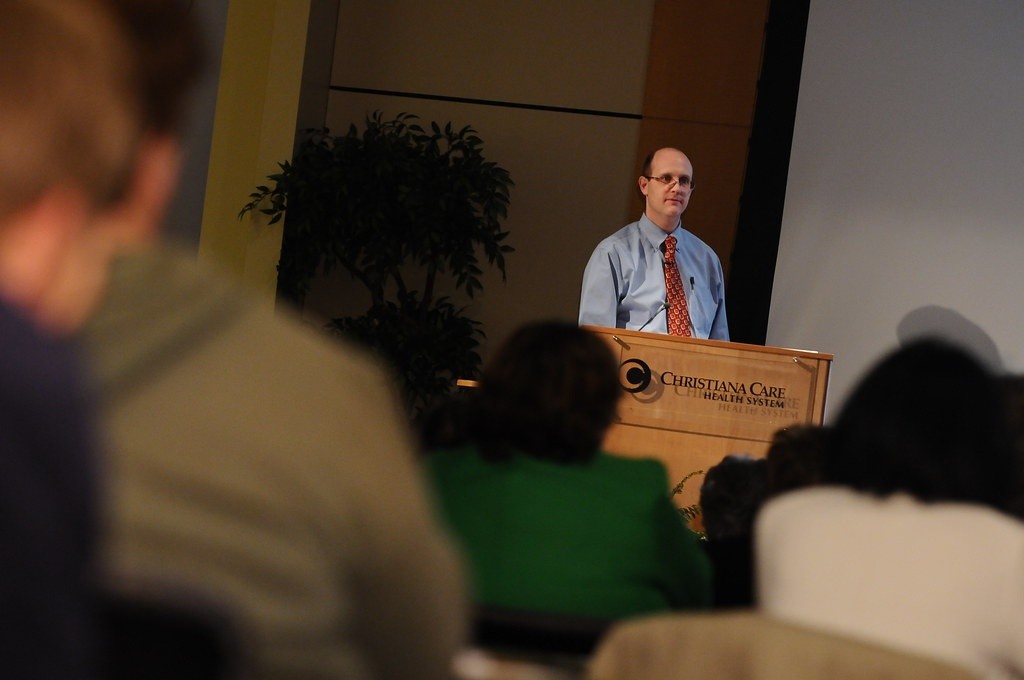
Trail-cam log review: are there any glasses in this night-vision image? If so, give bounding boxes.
[642,172,697,190]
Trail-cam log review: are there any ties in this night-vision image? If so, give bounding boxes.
[664,235,692,338]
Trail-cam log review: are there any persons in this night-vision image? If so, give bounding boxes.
[698,331,1024,679]
[421,324,710,624]
[0,0,209,680]
[578,148,731,343]
